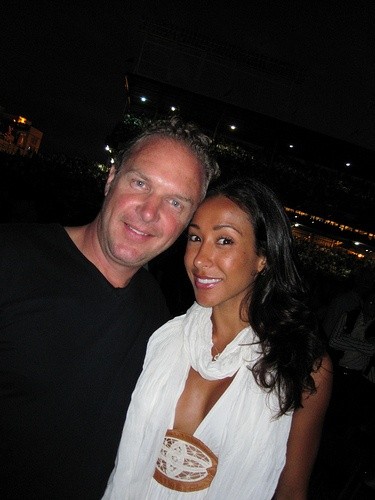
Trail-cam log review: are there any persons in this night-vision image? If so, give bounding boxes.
[0,112,223,500]
[101,174,333,500]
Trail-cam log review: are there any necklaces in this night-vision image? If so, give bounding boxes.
[211,341,228,361]
